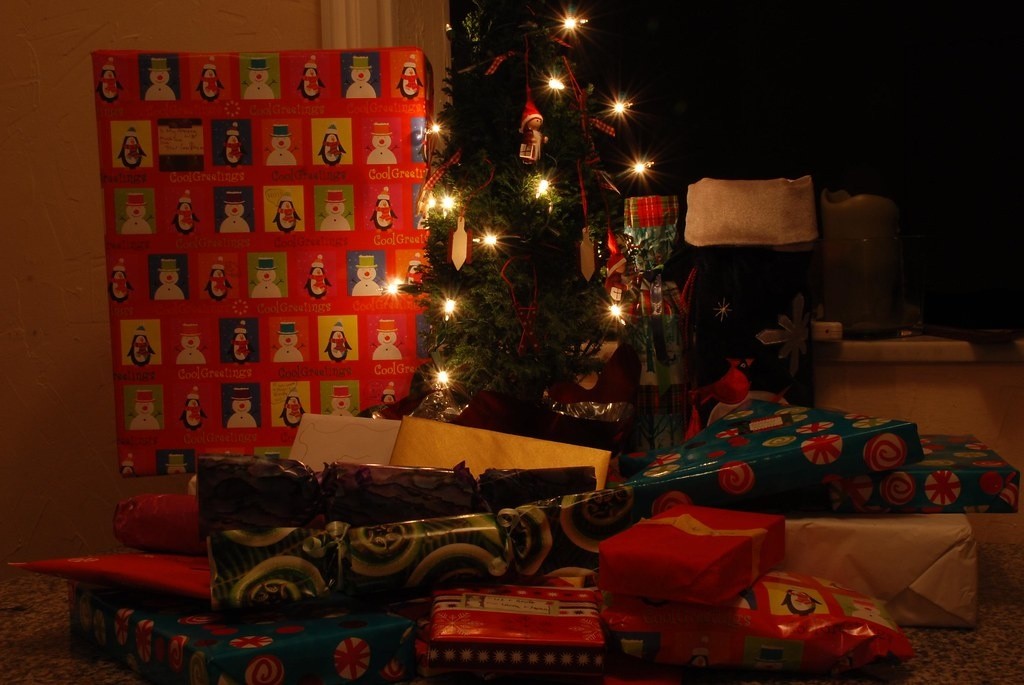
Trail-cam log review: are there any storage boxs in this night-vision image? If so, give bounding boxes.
[73,400,1019,685]
